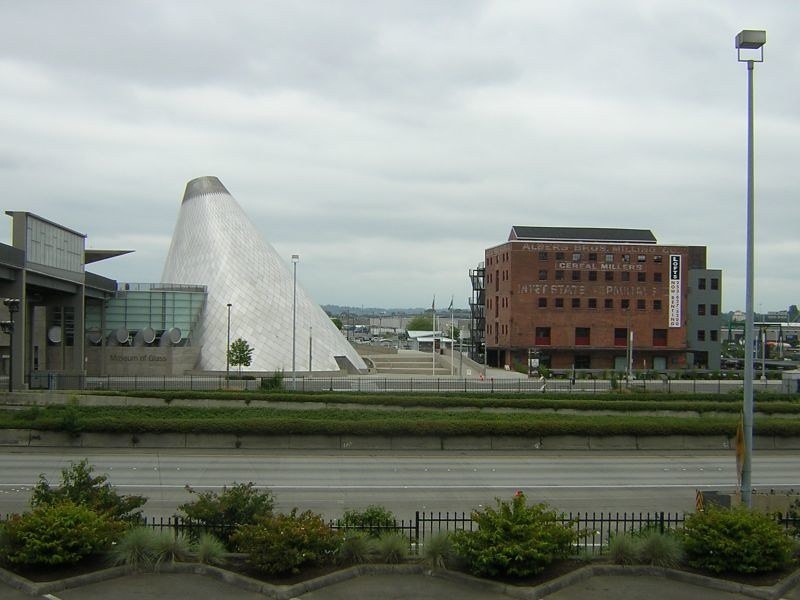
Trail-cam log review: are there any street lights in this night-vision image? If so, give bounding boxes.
[291,255,299,392]
[0,298,21,392]
[734,28,767,511]
[227,303,232,391]
